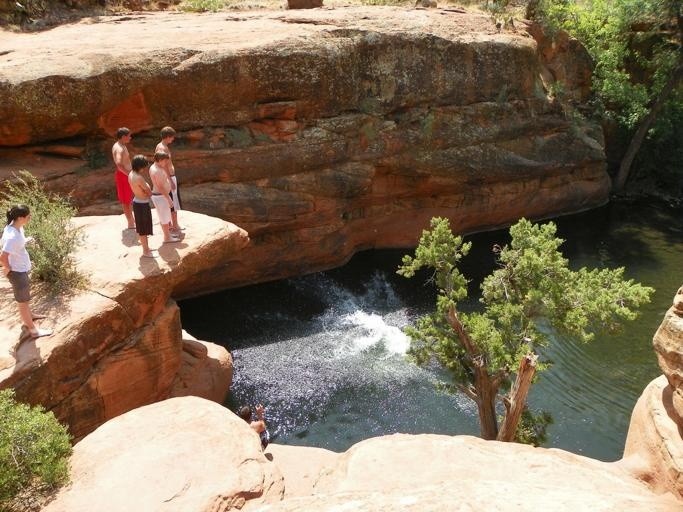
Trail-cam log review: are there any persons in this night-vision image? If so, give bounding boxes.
[238,403,271,453]
[0,203,54,338]
[128,154,161,258]
[111,127,138,229]
[148,150,181,243]
[154,126,186,230]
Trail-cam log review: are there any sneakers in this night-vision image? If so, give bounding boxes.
[168,225,186,230]
[143,252,159,258]
[163,234,180,242]
